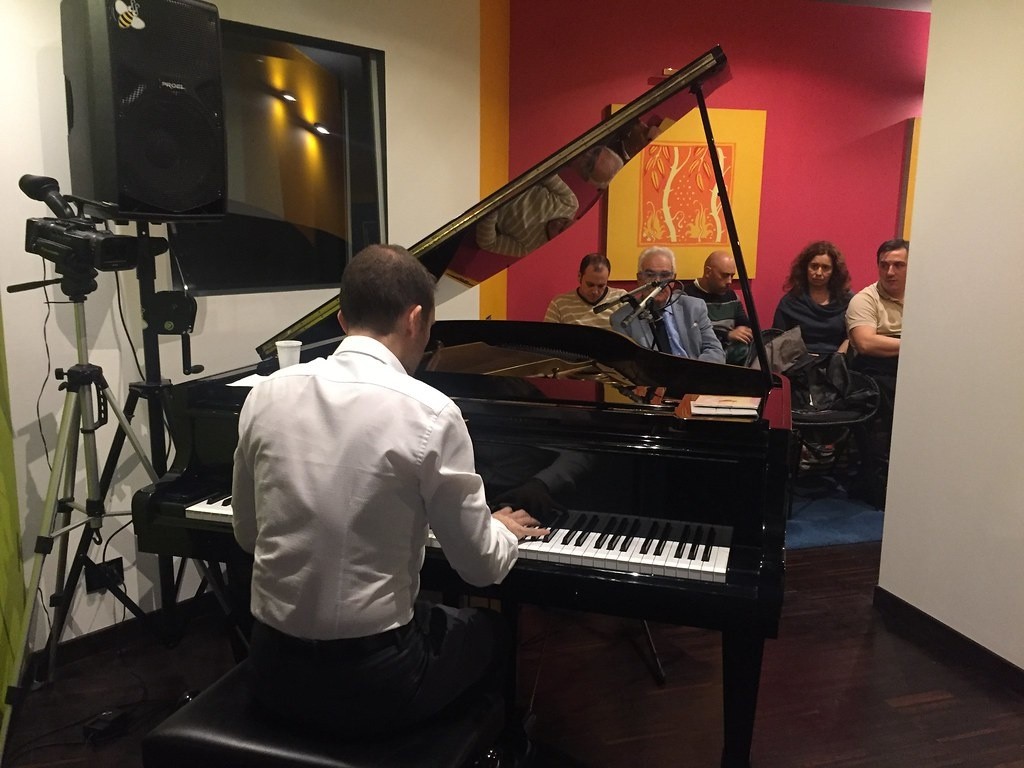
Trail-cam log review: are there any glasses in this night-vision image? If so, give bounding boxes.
[643,272,674,280]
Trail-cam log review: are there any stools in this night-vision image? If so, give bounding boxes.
[141,654,510,768]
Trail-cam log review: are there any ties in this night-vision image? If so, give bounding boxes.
[651,309,672,354]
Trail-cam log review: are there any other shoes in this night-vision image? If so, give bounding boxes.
[820,445,834,457]
[512,736,537,768]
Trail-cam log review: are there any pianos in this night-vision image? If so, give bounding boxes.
[129,45,799,768]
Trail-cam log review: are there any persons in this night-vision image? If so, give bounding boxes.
[233,244,550,755]
[545,253,632,327]
[846,239,910,365]
[571,117,660,188]
[610,246,755,368]
[773,241,855,357]
[477,175,579,257]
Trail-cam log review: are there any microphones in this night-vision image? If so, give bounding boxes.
[593,280,659,314]
[620,279,672,328]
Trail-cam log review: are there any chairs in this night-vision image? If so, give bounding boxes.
[745,328,880,520]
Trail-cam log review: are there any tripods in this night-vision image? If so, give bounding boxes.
[0,174,251,761]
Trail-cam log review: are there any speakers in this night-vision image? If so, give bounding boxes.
[58,0,228,219]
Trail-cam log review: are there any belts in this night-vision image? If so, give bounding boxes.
[250,620,417,655]
